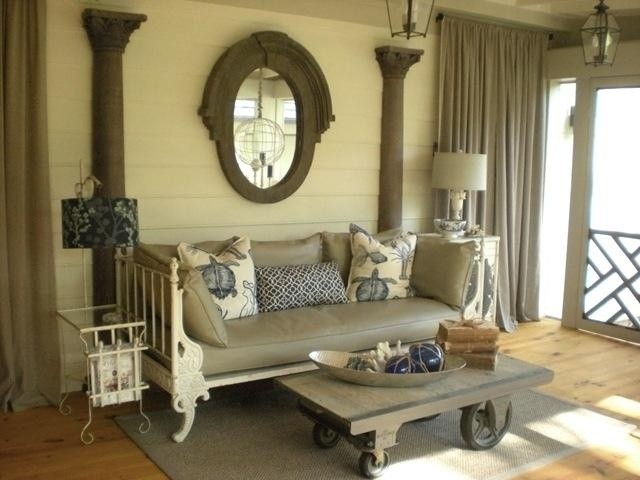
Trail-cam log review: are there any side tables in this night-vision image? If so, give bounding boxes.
[55,304,152,446]
[422,232,500,322]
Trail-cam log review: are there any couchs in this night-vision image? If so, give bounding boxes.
[103,223,486,442]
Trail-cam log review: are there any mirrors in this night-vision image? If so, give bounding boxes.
[198,31,337,204]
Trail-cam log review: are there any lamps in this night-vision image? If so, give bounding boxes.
[385,0,435,41]
[430,149,487,219]
[60,159,138,308]
[234,67,284,188]
[579,0,624,73]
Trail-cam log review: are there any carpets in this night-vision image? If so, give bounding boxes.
[112,389,636,480]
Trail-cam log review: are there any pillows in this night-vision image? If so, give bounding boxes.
[254,260,351,314]
[140,240,234,259]
[177,236,259,321]
[250,232,324,266]
[134,238,228,349]
[411,235,476,311]
[345,223,417,303]
[322,229,402,287]
